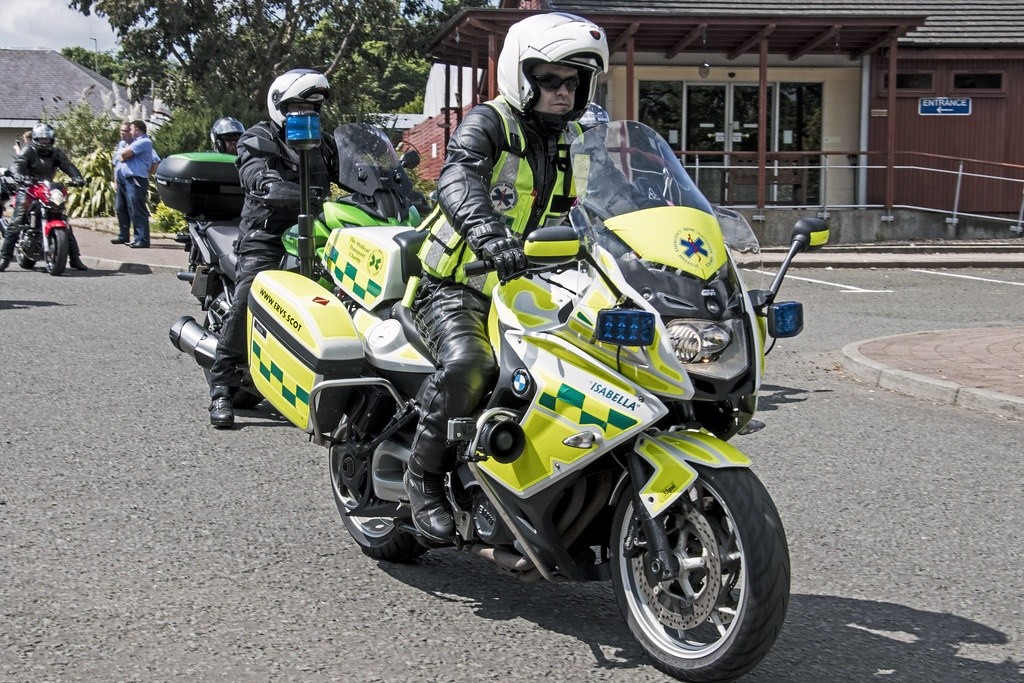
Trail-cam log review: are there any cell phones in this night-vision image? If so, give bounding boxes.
[16,140,20,146]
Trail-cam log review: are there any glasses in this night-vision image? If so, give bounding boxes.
[532,75,580,92]
[119,130,131,135]
[217,134,241,142]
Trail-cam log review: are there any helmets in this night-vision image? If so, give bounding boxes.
[210,116,245,153]
[497,11,610,121]
[32,123,55,155]
[267,69,331,128]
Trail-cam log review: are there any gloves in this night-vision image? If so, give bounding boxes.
[467,222,533,286]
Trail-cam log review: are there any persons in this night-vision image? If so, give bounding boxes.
[110,120,162,248]
[0,123,88,273]
[210,117,245,155]
[208,69,358,426]
[401,12,648,544]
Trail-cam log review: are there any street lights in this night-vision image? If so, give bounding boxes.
[89,36,99,72]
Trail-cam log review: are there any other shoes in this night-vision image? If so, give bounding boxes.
[0,258,9,272]
[69,257,88,270]
[110,237,130,244]
[131,241,149,248]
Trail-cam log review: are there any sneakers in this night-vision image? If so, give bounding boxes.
[208,397,234,426]
[403,455,458,544]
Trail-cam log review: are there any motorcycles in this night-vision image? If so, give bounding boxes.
[246,108,830,683]
[153,122,434,409]
[0,167,92,276]
